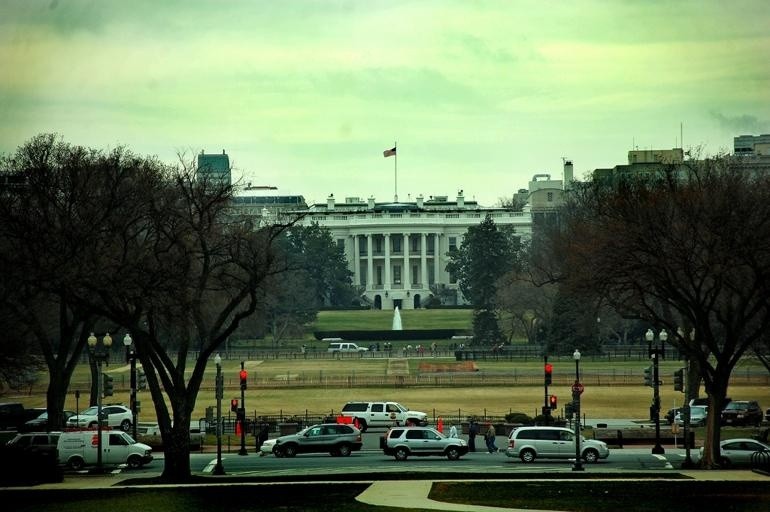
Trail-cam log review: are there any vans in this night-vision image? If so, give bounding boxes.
[6,431,61,461]
[148,419,208,448]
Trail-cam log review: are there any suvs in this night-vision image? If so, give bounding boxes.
[507,425,610,463]
[276,422,364,458]
[721,400,764,427]
[379,427,470,460]
[67,406,133,431]
[665,397,732,425]
[323,416,342,424]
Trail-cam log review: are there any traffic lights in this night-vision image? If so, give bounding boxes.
[644,367,651,386]
[551,396,557,409]
[231,399,238,411]
[137,369,146,391]
[674,371,682,390]
[104,375,114,397]
[544,364,551,385]
[240,371,247,390]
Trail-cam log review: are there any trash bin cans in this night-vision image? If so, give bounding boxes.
[279,423,298,437]
[189,429,201,450]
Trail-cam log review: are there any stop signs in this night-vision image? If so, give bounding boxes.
[571,382,585,395]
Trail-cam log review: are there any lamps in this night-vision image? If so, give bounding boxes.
[385,290,389,299]
[407,290,410,298]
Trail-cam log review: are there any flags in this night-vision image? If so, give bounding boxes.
[384,148,395,159]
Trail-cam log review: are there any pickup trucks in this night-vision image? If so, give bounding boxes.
[327,341,369,356]
[56,430,154,469]
[0,403,48,429]
[341,400,429,432]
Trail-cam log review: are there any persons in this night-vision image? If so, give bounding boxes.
[406,418,500,455]
[369,337,470,358]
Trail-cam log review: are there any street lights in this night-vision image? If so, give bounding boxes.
[124,334,137,442]
[597,316,602,342]
[213,354,225,474]
[573,350,584,470]
[676,327,695,467]
[88,331,113,471]
[645,329,667,455]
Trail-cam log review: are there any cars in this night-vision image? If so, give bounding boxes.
[765,408,770,422]
[25,410,77,427]
[674,404,708,426]
[260,428,342,456]
[697,437,770,468]
